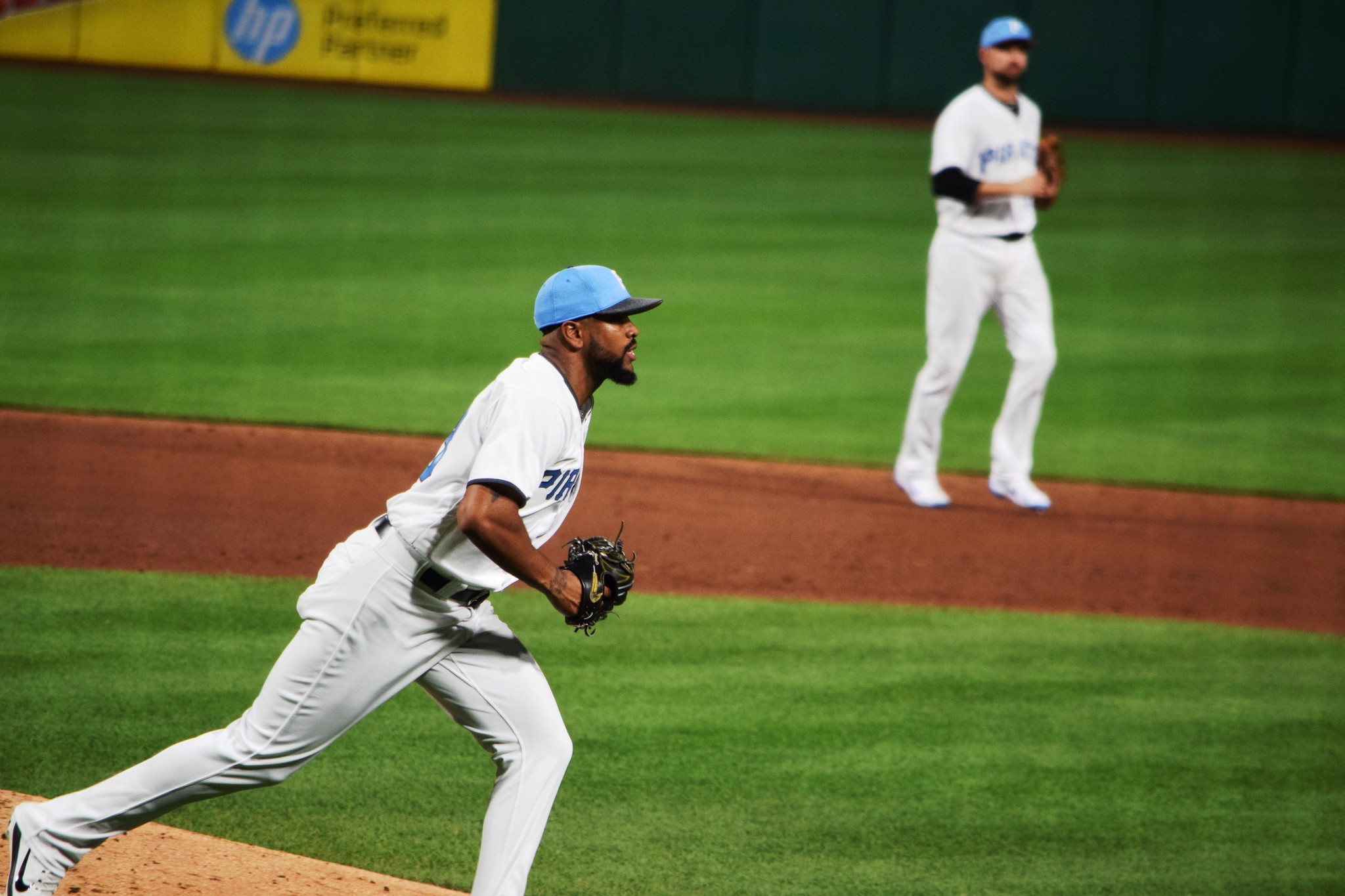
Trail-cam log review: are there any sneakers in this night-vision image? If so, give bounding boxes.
[4,800,67,896]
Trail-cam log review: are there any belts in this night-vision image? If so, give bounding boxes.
[995,230,1026,242]
[374,515,490,608]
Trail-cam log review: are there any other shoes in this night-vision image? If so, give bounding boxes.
[986,472,1050,510]
[893,466,949,507]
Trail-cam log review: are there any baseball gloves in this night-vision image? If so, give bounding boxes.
[1035,133,1062,203]
[563,535,634,639]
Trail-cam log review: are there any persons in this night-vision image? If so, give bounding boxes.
[892,19,1062,508]
[6,264,664,896]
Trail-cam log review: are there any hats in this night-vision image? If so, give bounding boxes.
[980,16,1031,47]
[533,264,663,331]
